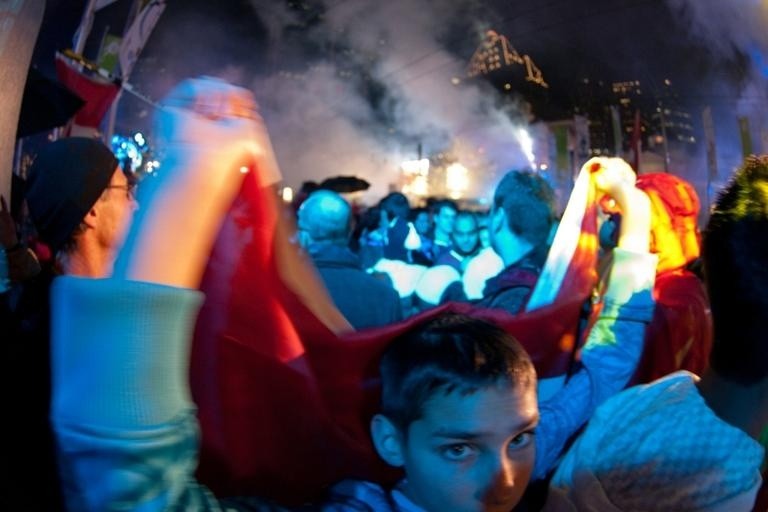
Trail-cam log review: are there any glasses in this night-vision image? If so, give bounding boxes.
[103,183,137,203]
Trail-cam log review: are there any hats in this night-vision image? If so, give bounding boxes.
[23,135,121,249]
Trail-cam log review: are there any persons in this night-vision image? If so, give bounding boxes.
[543,147,766,512]
[49,71,660,511]
[282,167,713,392]
[0,132,140,511]
